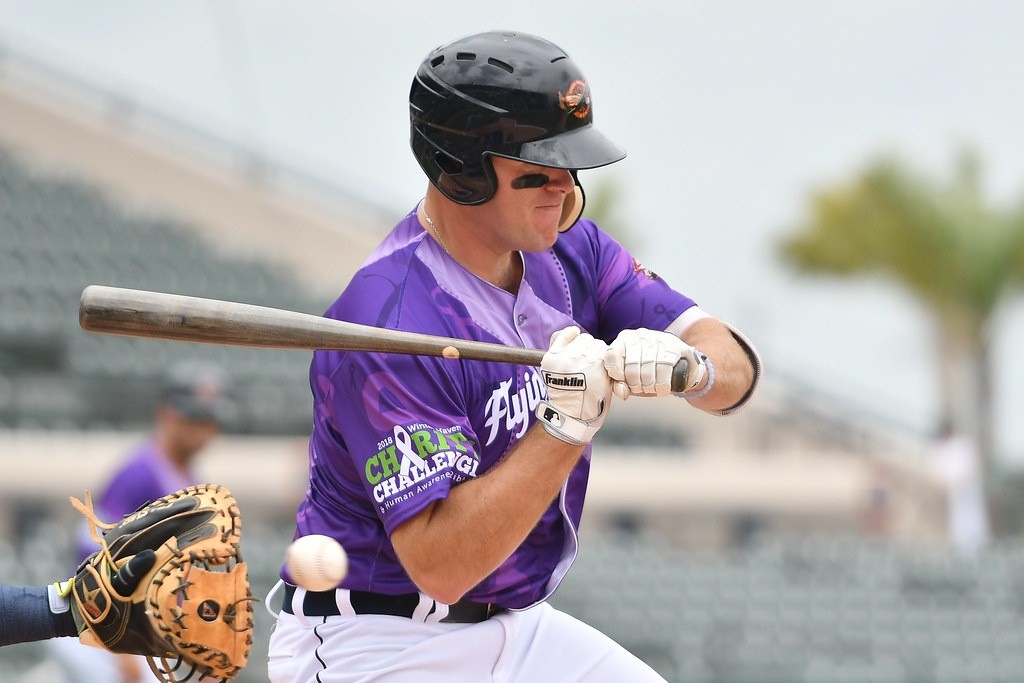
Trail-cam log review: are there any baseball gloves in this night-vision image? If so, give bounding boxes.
[64,479,261,683]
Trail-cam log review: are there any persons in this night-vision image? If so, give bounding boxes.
[65,357,244,683]
[266,30,762,683]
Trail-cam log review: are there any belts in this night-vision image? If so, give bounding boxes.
[278,583,507,623]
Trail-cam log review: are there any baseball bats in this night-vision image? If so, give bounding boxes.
[77,284,690,392]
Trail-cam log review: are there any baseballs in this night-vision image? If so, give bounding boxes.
[285,534,350,594]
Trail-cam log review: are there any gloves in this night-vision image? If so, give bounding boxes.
[535,327,613,446]
[603,327,705,402]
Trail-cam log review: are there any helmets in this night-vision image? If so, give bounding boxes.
[408,32,626,207]
